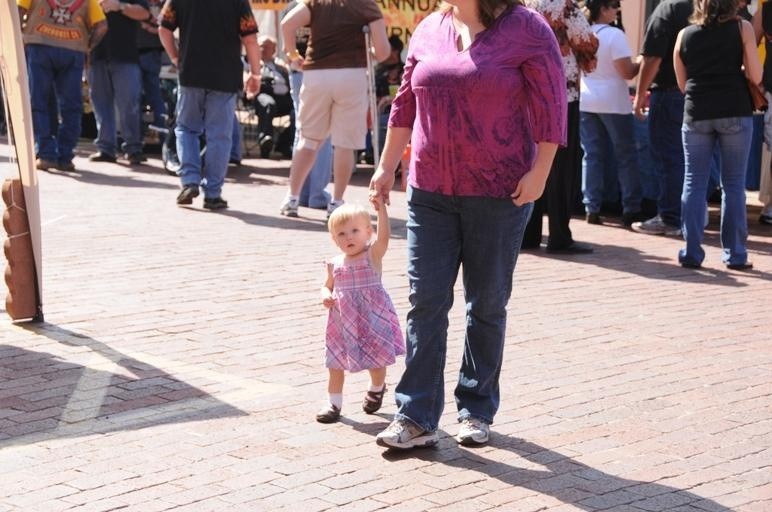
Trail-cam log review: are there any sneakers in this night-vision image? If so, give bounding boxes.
[36,158,57,169]
[457,415,490,445]
[280,198,301,217]
[58,161,75,172]
[630,214,681,236]
[759,206,772,223]
[374,415,439,450]
[175,185,200,205]
[204,196,228,208]
[325,199,344,217]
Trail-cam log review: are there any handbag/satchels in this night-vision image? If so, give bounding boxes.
[748,74,769,111]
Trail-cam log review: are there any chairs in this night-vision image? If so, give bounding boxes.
[241,91,293,157]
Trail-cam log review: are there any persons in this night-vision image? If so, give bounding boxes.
[366,0,569,453]
[314,191,405,424]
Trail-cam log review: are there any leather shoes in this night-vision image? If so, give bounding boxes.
[125,149,148,163]
[89,150,117,162]
[274,142,292,158]
[586,212,603,225]
[316,402,342,424]
[625,212,653,227]
[546,236,594,255]
[362,381,386,413]
[727,258,754,270]
[259,134,273,157]
